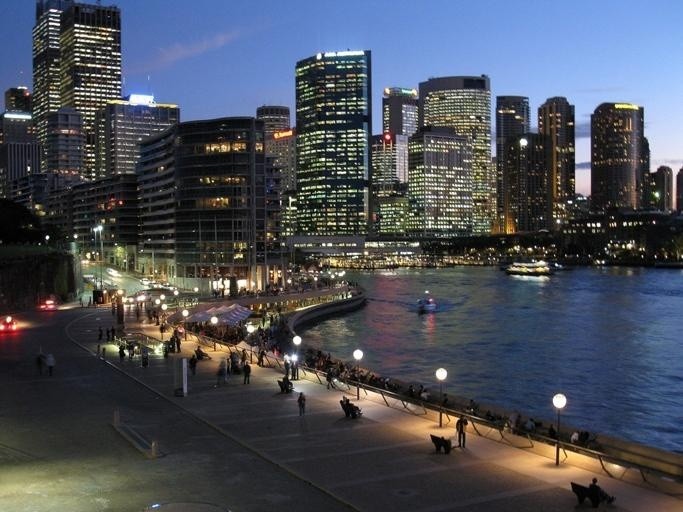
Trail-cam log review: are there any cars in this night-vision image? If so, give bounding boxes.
[0,316,16,332]
[81,268,163,288]
[36,298,58,311]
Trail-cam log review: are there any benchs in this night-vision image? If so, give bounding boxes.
[194,349,203,360]
[340,400,357,419]
[278,379,293,394]
[571,481,613,511]
[430,434,452,451]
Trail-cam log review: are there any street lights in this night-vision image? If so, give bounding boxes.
[352,348,362,400]
[117,286,198,340]
[93,227,97,290]
[551,393,566,465]
[268,268,346,293]
[97,225,104,289]
[435,366,447,428]
[292,335,302,379]
[210,316,218,351]
[246,325,254,363]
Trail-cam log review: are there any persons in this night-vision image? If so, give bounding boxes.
[298,392,306,416]
[589,478,616,504]
[136,303,298,381]
[243,362,251,385]
[98,325,115,340]
[216,360,228,385]
[119,343,134,360]
[35,352,55,376]
[283,373,293,387]
[302,348,590,448]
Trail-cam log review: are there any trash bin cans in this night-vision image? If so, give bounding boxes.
[93,289,108,304]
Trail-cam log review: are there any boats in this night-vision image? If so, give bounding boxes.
[506,259,553,275]
[415,299,437,312]
